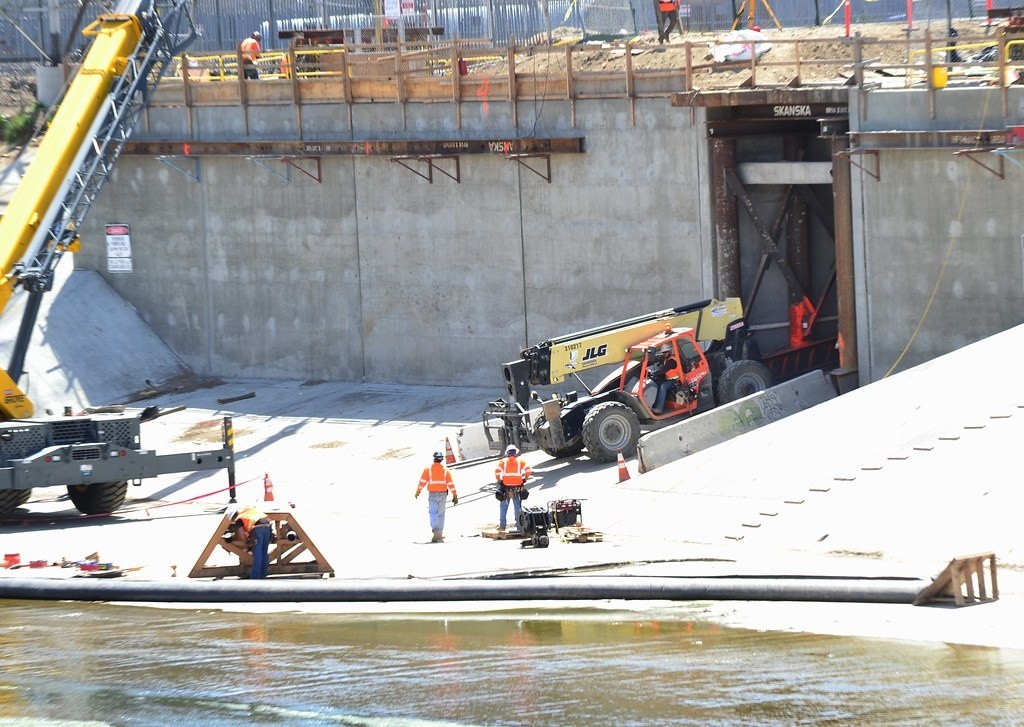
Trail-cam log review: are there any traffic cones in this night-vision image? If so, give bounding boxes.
[262,472,275,502]
[445,436,457,465]
[613,448,631,485]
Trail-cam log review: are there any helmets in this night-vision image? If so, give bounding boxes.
[224,504,239,521]
[505,445,520,456]
[433,452,443,459]
[659,345,671,352]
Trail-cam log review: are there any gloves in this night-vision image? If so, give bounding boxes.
[416,493,419,498]
[452,495,459,506]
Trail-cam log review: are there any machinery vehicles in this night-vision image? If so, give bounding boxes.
[0,0,237,523]
[480,116,838,465]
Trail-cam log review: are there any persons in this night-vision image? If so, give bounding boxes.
[649,345,680,413]
[224,505,271,578]
[495,445,531,531]
[241,31,263,79]
[415,451,457,542]
[658,0,681,43]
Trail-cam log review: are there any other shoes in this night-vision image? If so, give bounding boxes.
[497,527,506,530]
[651,407,663,414]
[432,530,442,543]
